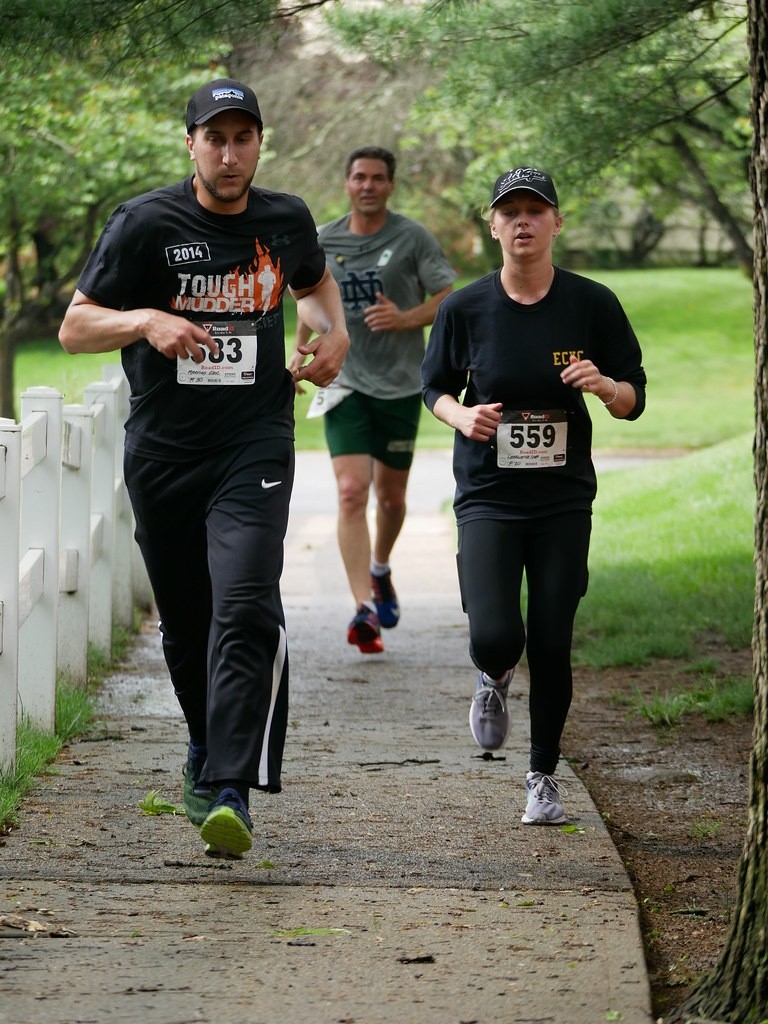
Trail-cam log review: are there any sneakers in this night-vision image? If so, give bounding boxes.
[201,788,253,862]
[371,572,401,629]
[521,770,569,824]
[469,669,515,751]
[347,604,385,653]
[182,738,213,827]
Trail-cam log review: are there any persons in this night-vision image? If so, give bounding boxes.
[58,78,352,858]
[288,146,462,655]
[421,167,648,824]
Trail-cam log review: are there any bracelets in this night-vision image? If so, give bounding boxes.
[600,374,618,405]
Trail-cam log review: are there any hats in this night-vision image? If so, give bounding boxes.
[491,167,559,210]
[187,78,264,132]
